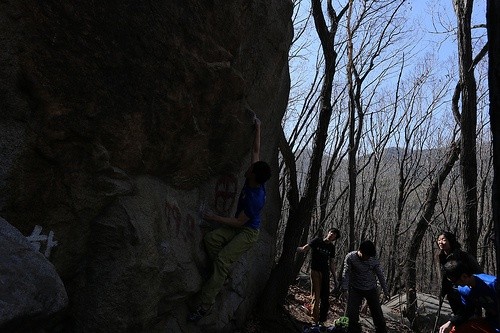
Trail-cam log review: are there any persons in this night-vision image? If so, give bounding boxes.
[436,231,500,333]
[296,228,341,327]
[339,240,391,333]
[185,118,272,324]
